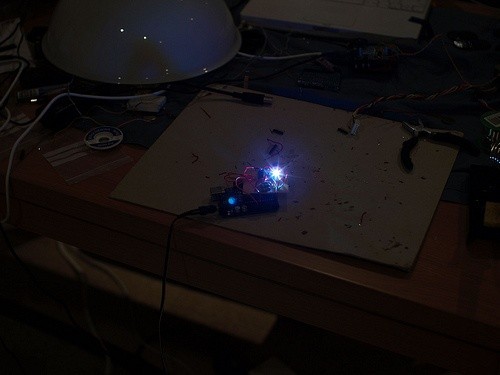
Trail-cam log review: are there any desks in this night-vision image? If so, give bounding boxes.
[1,26,500,374]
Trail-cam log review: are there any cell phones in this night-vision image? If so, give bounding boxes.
[217,181,279,217]
